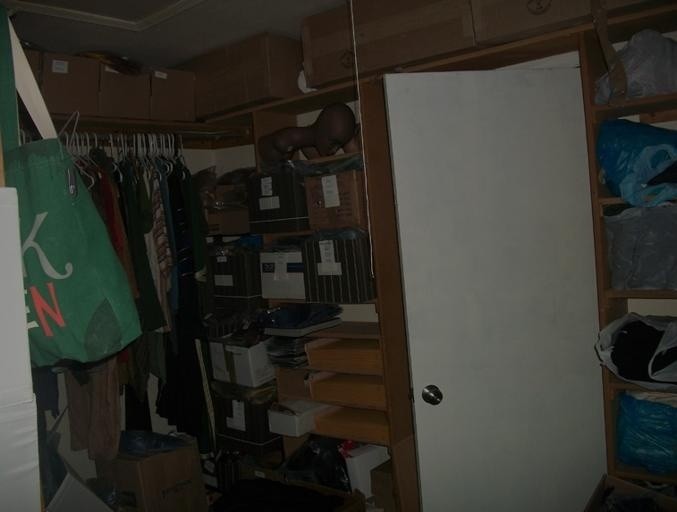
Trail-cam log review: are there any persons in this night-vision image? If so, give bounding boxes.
[258,101,355,163]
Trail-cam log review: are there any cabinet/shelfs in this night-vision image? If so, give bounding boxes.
[571,2,676,484]
[189,73,420,512]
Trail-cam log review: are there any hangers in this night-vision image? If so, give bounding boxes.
[15,127,189,198]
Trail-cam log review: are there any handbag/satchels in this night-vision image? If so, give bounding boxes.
[0,28,146,373]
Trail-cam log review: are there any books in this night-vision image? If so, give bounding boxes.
[264,317,341,339]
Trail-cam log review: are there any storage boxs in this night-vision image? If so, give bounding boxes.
[580,471,676,511]
[89,434,209,510]
[19,31,299,122]
